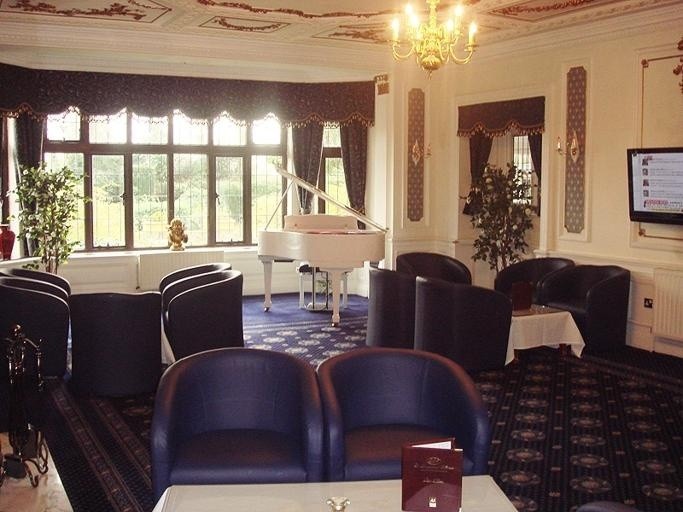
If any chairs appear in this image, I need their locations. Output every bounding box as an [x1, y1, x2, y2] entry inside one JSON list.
[[1, 261, 245, 400], [151, 346, 321, 499], [317, 347, 490, 482], [366, 250, 631, 375]]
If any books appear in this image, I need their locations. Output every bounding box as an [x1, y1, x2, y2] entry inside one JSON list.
[[400, 438, 463, 511]]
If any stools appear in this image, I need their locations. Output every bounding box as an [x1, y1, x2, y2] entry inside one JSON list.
[[297, 261, 349, 309]]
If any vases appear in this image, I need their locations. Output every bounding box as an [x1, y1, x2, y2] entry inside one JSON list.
[[0, 223, 17, 260]]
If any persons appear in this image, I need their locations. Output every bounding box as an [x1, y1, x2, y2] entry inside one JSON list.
[[167, 218, 188, 247]]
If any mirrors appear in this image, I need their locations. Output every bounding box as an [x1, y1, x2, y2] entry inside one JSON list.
[[475, 132, 539, 212]]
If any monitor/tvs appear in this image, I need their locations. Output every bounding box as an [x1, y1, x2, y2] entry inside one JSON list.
[[626, 147, 683, 224]]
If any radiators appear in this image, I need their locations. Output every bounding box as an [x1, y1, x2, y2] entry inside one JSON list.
[[653, 263, 683, 346]]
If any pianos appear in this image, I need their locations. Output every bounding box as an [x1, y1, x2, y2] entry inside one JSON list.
[[257, 163, 387, 327]]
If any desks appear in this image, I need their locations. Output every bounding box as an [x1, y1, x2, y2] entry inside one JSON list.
[[150, 474, 518, 512]]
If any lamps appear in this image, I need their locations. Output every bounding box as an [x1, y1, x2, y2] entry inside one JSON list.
[[385, 0, 479, 80], [407, 142, 432, 163], [551, 135, 578, 156]]
